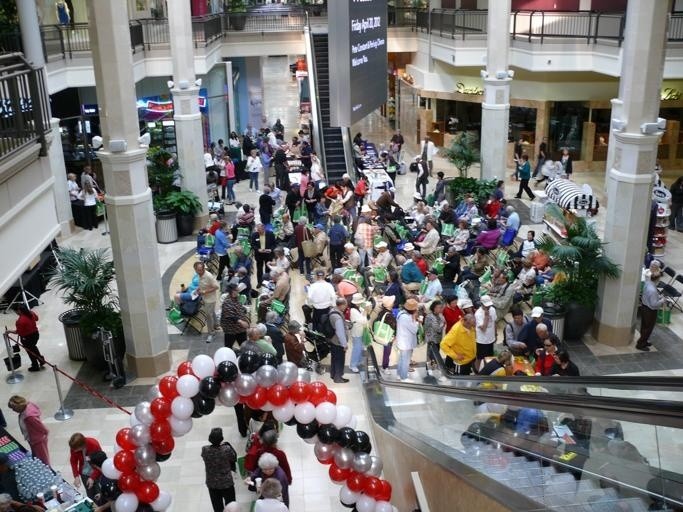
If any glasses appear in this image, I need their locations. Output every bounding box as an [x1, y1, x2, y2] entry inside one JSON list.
[[543, 344, 552, 347]]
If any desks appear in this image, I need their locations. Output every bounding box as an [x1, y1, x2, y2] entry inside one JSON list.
[[0, 431, 95, 511]]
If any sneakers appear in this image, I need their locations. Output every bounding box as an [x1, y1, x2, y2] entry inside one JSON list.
[[635, 341, 652, 351], [349, 365, 359, 372], [206, 335, 216, 342]]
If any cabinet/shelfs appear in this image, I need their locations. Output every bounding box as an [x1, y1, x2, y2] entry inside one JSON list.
[[362, 140, 393, 213]]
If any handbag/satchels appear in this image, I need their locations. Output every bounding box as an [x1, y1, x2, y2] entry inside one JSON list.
[[420, 283, 428, 295], [388, 225, 408, 244], [374, 320, 394, 347], [248, 475, 263, 493], [271, 299, 285, 315], [206, 226, 251, 263], [417, 326, 425, 346], [363, 326, 371, 346], [657, 306, 671, 325], [236, 456, 251, 477], [441, 223, 456, 237], [497, 343, 509, 357], [169, 305, 184, 325], [320, 311, 336, 339], [479, 250, 510, 296], [292, 207, 309, 223], [432, 259, 444, 274], [302, 239, 316, 257], [238, 294, 248, 304]]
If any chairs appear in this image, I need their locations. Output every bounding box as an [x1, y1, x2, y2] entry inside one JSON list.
[[652, 259, 683, 313]]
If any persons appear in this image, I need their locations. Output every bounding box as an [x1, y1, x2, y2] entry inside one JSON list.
[[65, 0, 74, 33], [55, 0, 72, 38], [0, 115, 683, 512]]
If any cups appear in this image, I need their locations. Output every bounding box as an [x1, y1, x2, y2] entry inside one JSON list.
[[419, 316, 424, 320], [254, 477, 262, 487], [36, 492, 47, 505]]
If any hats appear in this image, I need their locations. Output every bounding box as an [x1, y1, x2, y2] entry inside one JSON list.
[[531, 306, 544, 318], [351, 293, 364, 305], [344, 266, 387, 285], [480, 295, 493, 307], [288, 320, 301, 332], [413, 192, 423, 200], [314, 224, 325, 230], [375, 241, 388, 248], [382, 295, 396, 309], [403, 243, 414, 251], [403, 299, 418, 311], [361, 204, 372, 212], [461, 299, 474, 310]]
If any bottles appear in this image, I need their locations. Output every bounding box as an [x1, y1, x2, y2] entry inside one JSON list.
[[13, 456, 63, 503]]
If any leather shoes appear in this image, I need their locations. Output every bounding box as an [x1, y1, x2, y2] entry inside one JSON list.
[[335, 377, 349, 383]]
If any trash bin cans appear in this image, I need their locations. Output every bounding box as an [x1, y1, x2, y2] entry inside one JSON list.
[[58, 309, 87, 363], [154, 208, 178, 244]]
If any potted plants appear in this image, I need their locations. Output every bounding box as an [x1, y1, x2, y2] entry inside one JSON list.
[[538, 200, 620, 341], [443, 127, 497, 209], [145, 146, 203, 243], [228, 7, 247, 30], [41, 246, 116, 361]]
